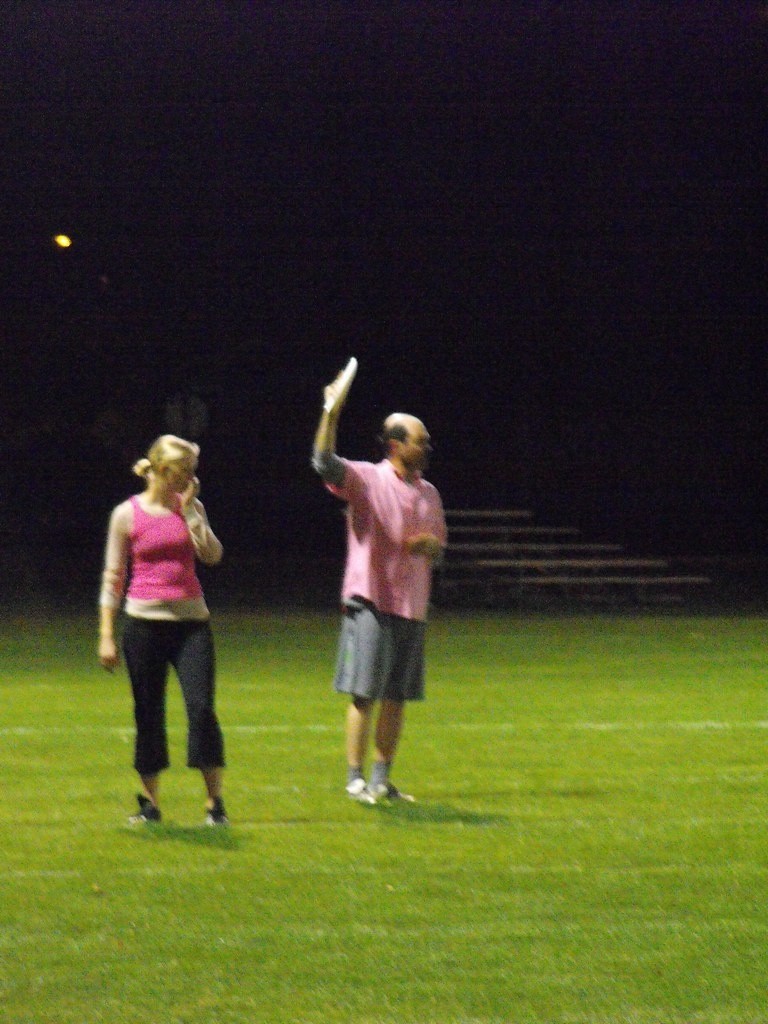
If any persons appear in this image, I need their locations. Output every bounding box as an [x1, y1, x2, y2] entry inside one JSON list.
[[97, 435, 230, 825], [313, 371, 446, 809]]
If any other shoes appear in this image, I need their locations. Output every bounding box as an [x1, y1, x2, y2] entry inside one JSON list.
[[346, 778, 377, 805], [206, 808, 228, 827], [367, 779, 414, 803], [127, 794, 160, 830]]
